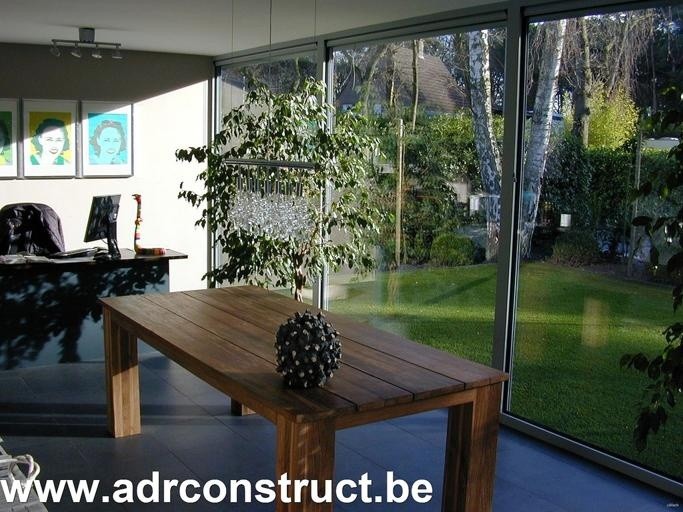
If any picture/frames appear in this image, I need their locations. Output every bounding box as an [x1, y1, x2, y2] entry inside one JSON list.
[[0, 95, 134, 180]]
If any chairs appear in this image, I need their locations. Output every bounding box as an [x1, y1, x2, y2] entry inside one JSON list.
[[0, 203, 62, 255]]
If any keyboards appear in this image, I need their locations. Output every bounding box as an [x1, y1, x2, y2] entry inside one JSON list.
[[52, 248, 95, 258]]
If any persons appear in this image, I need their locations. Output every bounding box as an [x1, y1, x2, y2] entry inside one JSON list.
[[89, 119, 128, 164], [30, 118, 70, 165]]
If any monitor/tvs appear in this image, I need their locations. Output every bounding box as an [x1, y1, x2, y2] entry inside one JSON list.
[[85, 194, 121, 259]]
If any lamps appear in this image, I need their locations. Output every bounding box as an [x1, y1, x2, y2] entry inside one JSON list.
[[49, 27, 123, 59], [225, 0, 320, 241]]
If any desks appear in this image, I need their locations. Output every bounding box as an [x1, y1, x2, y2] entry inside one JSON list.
[[99, 284, 510, 512], [0, 248, 188, 371]]
